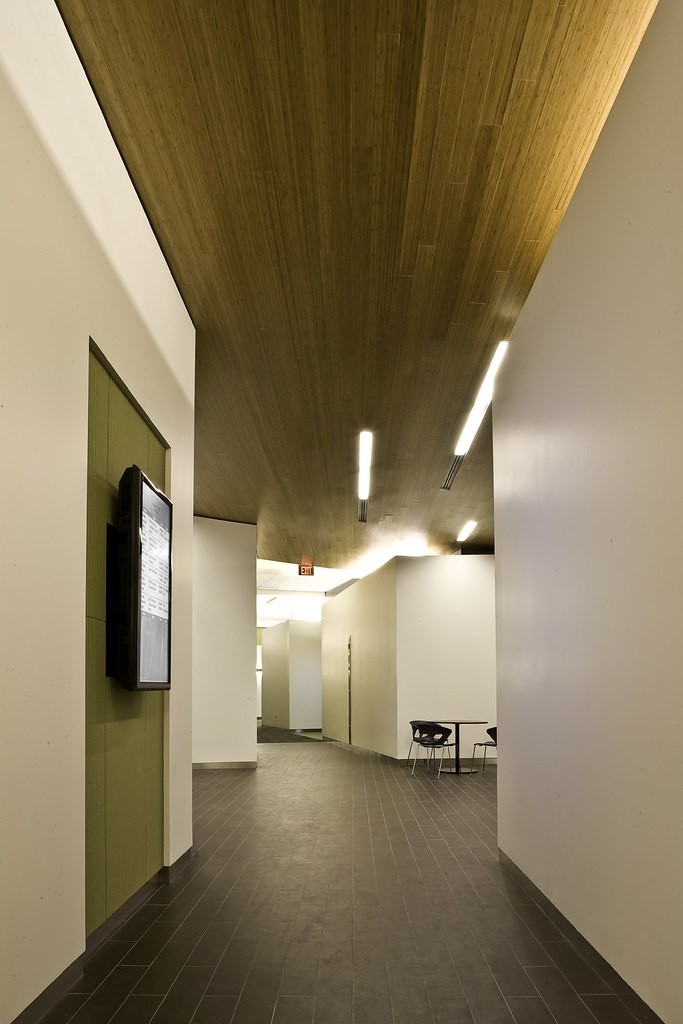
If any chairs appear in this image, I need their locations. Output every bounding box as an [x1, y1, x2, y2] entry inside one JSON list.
[[470, 727, 497, 776], [406, 720, 463, 779]]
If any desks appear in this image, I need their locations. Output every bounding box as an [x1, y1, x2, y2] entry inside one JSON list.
[[428, 721, 488, 774]]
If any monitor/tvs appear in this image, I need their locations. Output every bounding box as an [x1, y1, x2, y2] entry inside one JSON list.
[[113, 464, 173, 692]]
